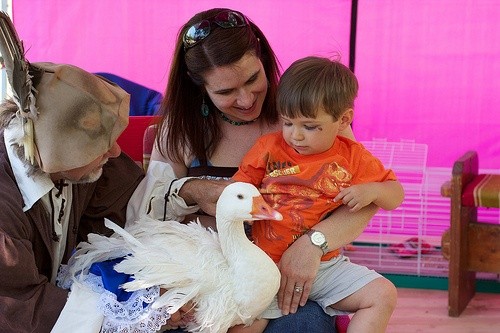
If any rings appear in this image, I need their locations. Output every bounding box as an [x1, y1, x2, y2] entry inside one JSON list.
[[294, 286, 303, 293]]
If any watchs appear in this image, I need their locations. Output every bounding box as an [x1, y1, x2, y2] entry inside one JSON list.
[[303, 229, 329, 256]]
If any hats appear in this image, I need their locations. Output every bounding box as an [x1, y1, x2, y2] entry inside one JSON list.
[[0, 12, 130, 172]]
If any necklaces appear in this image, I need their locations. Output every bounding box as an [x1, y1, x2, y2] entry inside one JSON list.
[[219, 112, 260, 126]]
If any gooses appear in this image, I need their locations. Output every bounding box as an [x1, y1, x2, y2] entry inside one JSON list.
[[58, 181, 284, 333]]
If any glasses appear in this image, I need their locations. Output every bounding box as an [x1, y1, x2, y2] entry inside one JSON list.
[[181, 10, 250, 51]]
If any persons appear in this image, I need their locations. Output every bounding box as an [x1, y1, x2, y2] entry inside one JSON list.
[[0, 12, 235, 333], [230, 56, 404, 333], [148, 7, 379, 333]]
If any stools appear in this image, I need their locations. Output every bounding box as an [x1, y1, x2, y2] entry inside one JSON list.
[[442, 150, 500, 317]]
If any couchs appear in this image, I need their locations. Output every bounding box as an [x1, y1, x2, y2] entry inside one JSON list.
[[116, 115, 351, 333]]
